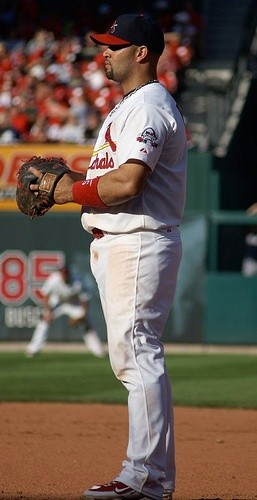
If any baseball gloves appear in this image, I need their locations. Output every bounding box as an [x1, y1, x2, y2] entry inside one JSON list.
[[15, 155, 69, 219]]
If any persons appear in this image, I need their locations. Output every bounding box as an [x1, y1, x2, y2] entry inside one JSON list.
[[30, 14, 188, 500], [26, 264, 105, 360], [0, 0, 201, 145]]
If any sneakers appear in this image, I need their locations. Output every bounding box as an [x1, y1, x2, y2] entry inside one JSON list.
[[83, 480, 172, 500]]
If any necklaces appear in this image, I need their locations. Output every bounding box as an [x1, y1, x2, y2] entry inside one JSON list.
[[109, 79, 159, 118]]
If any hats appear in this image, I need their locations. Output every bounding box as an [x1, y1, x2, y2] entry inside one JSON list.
[[90, 14, 165, 55]]
